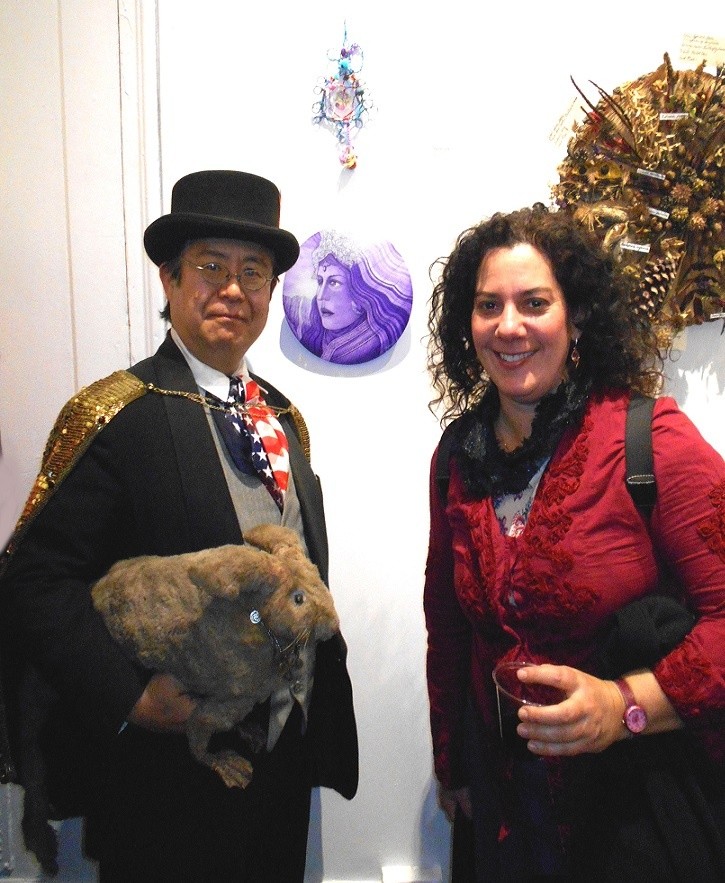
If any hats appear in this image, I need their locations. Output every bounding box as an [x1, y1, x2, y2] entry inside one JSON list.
[[143, 169, 301, 277]]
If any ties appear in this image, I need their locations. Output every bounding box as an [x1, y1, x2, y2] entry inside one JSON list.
[[227, 376, 286, 509]]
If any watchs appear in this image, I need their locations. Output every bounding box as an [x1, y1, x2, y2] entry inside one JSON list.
[[613, 679, 648, 737]]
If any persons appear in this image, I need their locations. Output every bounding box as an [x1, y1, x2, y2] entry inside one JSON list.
[[422, 203, 725, 882], [0, 169, 361, 883]]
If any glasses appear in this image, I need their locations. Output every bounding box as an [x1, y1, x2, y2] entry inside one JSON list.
[[178, 251, 277, 292]]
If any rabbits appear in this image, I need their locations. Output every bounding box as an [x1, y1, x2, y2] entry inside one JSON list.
[[16, 523, 340, 880]]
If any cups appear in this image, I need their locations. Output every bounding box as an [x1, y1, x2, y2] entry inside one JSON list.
[[491, 661, 567, 753]]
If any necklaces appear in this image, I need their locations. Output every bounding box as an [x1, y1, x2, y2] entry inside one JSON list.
[[494, 423, 511, 453]]
